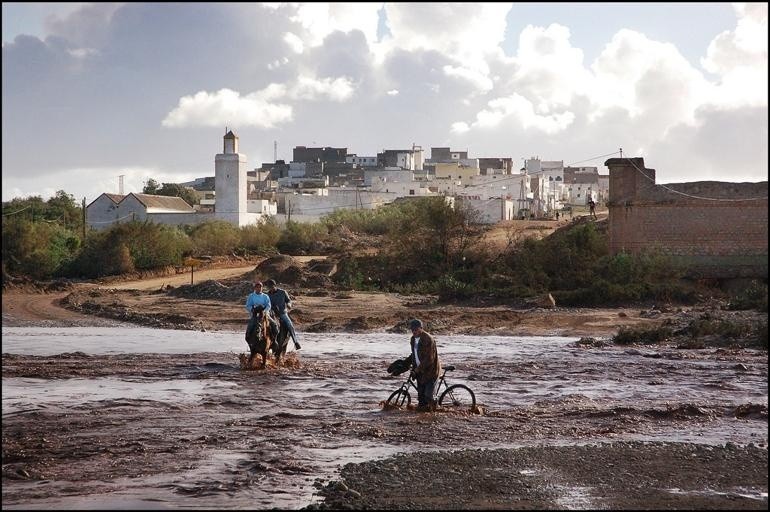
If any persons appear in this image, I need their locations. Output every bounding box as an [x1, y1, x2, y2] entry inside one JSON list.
[[246, 281, 279, 346], [587, 197, 595, 216], [556, 211, 559, 219], [266, 278, 301, 350], [404, 318, 441, 407]]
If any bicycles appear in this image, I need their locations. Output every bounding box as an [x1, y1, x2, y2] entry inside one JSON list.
[[383, 364, 477, 414]]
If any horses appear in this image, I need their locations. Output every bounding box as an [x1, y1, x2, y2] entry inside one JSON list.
[[245, 304, 280, 368], [271, 304, 291, 366]]
